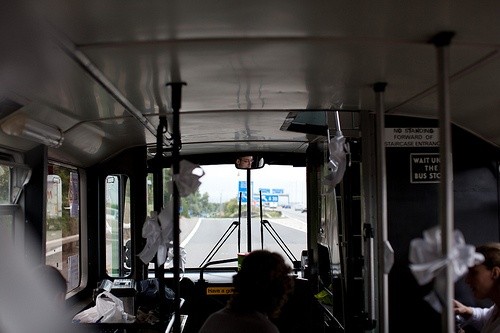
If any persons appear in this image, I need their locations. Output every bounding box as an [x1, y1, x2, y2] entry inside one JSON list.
[[198, 251, 294, 333], [0, 263, 103, 333], [454, 242, 500, 333], [236, 156, 253, 168]]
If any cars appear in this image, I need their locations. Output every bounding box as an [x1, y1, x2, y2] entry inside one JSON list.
[[264, 201, 307, 213]]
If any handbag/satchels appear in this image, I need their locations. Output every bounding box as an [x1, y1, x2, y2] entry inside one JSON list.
[[73, 291, 137, 323]]
